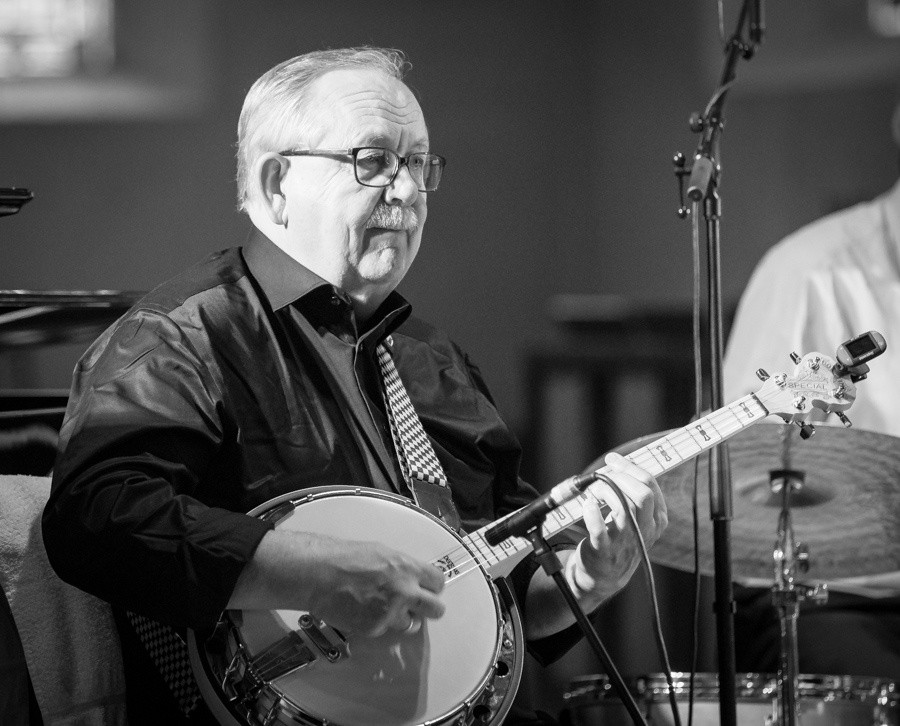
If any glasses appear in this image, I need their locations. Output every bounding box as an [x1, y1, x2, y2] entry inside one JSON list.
[[278, 146, 447, 192]]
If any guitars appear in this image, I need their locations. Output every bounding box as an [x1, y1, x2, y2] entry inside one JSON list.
[[177, 325, 893, 725]]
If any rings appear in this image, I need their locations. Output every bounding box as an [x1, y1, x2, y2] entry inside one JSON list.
[[401, 616, 414, 634]]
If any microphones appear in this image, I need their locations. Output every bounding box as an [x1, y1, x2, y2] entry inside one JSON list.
[[485, 473, 597, 546]]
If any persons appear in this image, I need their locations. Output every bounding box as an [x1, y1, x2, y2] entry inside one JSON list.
[[715, 178, 900, 680], [41, 46, 668, 726]]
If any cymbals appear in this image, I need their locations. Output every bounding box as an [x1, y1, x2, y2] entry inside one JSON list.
[[580, 416, 898, 581]]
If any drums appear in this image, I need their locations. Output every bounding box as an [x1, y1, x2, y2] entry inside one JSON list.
[[567, 666, 894, 726]]
